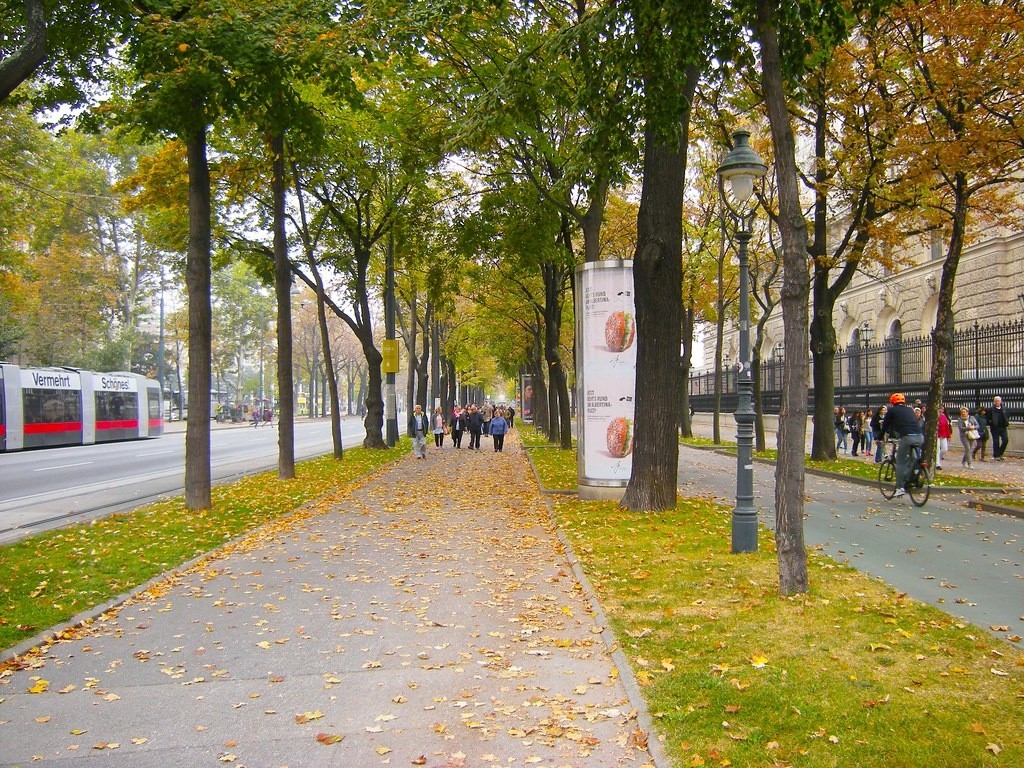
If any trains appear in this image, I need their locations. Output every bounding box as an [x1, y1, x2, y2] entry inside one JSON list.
[[0, 360, 168, 453]]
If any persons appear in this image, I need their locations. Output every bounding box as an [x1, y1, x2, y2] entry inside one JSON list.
[[431, 407, 445, 450], [688, 403, 694, 423], [215, 402, 274, 429], [525, 383, 534, 410], [450, 402, 514, 453], [360, 405, 366, 420], [832, 393, 1010, 496], [408, 405, 428, 459]]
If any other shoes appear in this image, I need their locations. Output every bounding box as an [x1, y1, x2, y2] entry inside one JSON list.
[[837, 452, 840, 454], [844, 452, 847, 454], [959, 462, 964, 468], [417, 457, 421, 460], [936, 466, 942, 470], [422, 454, 426, 459], [457, 446, 461, 449], [852, 451, 858, 456], [441, 445, 444, 449], [874, 461, 882, 465], [468, 445, 474, 450], [495, 448, 502, 452], [436, 447, 439, 450], [454, 444, 456, 447], [979, 456, 988, 462], [966, 465, 975, 470], [992, 456, 1004, 462], [484, 435, 488, 437], [865, 451, 872, 456]]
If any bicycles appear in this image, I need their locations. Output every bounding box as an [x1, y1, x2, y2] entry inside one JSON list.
[[876, 438, 930, 508]]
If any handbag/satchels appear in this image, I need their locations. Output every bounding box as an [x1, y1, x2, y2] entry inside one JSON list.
[[967, 429, 980, 440], [443, 426, 449, 434], [844, 425, 850, 433]]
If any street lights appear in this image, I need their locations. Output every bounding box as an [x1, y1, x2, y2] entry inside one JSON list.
[[861, 322, 873, 412], [723, 354, 732, 412], [715, 127, 768, 553], [689, 364, 694, 415], [776, 343, 785, 397]]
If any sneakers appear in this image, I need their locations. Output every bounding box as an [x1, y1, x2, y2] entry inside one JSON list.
[[892, 487, 906, 497]]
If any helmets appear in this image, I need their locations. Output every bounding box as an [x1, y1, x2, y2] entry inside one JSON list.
[[889, 393, 905, 405]]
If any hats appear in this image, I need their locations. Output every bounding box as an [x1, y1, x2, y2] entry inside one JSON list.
[[913, 406, 921, 412], [470, 406, 476, 410]]
